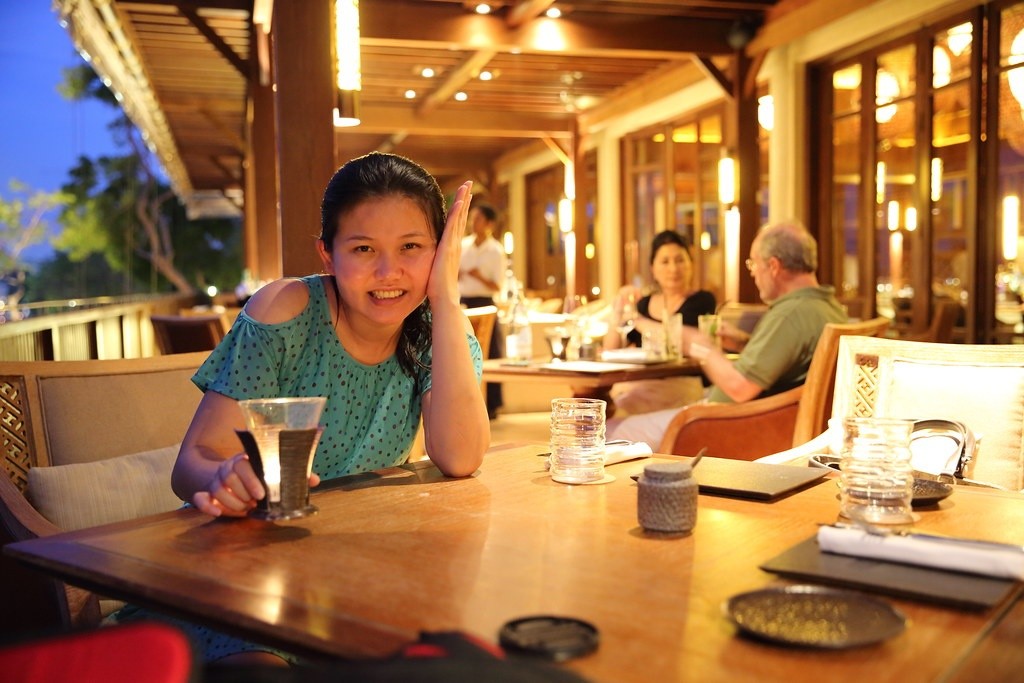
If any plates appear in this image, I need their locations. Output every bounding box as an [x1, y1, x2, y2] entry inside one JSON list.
[[728, 585, 907, 646]]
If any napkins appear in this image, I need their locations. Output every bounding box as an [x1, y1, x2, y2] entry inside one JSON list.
[[544, 441, 653, 470], [818, 524, 1024, 582]]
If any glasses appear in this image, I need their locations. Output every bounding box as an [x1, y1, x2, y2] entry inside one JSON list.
[[744, 254, 783, 271]]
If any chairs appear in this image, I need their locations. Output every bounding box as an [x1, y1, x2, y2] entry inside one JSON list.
[[462, 305, 497, 404], [0, 348, 255, 641], [715, 300, 772, 354], [660, 316, 891, 461], [147, 315, 224, 358], [752, 334, 1024, 493]]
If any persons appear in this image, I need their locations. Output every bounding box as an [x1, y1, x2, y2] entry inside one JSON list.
[[608, 215, 848, 455], [595, 230, 717, 438], [455, 202, 505, 422], [116, 153, 491, 683]]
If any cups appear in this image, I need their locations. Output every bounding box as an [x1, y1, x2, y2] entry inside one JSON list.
[[550, 396, 608, 483], [541, 310, 723, 364], [238, 396, 327, 516], [839, 418, 920, 532]]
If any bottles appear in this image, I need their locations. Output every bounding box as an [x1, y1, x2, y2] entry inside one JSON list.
[[504, 286, 533, 363], [636, 461, 699, 531]]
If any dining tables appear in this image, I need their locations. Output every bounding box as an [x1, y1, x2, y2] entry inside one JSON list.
[[0, 350, 1024, 683]]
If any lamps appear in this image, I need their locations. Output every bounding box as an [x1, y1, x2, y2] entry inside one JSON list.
[[332, 0, 362, 129], [557, 194, 572, 233], [504, 229, 514, 254]]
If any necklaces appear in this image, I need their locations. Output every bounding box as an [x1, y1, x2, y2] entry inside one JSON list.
[[663, 289, 687, 319]]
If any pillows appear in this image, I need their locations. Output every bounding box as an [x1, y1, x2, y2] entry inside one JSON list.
[[28, 442, 187, 618]]
